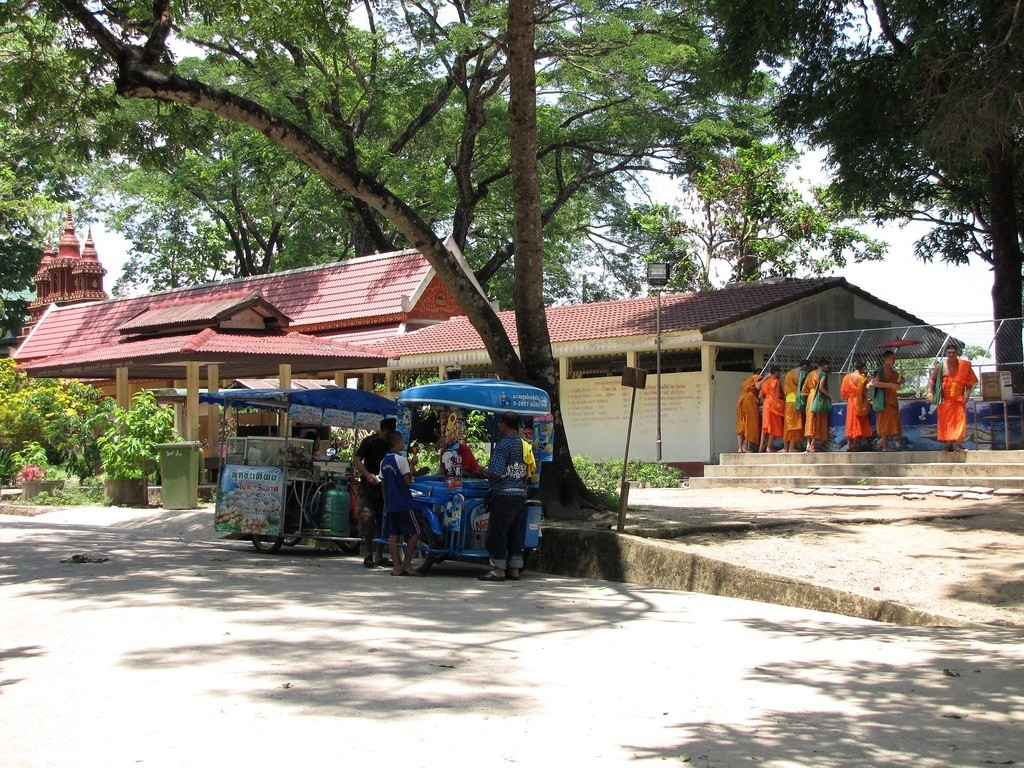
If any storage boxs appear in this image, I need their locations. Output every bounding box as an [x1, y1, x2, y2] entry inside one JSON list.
[[980, 370, 1014, 401]]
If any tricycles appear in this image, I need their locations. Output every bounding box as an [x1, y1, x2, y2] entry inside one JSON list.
[[196, 386, 395, 556], [367, 377, 554, 576]]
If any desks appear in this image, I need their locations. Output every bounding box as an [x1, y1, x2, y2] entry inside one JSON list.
[[973, 395, 1024, 450]]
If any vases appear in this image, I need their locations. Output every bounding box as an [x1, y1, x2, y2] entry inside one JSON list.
[[20, 479, 65, 502]]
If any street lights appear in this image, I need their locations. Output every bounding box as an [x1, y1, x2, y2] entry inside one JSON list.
[[646, 261, 672, 465]]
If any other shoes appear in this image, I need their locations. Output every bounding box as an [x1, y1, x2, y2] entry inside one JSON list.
[[364, 557, 375, 566], [375, 557, 394, 568], [737, 443, 969, 453]]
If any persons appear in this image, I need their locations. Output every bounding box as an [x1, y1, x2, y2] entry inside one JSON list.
[[378, 429, 425, 578], [866, 351, 906, 452], [478, 410, 530, 582], [926, 345, 979, 452], [735, 359, 838, 453], [354, 417, 397, 568], [440, 443, 488, 477], [840, 359, 872, 451], [304, 432, 328, 461]]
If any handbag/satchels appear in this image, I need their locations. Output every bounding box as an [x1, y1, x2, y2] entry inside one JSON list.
[[854, 374, 871, 416], [868, 367, 885, 411], [484, 485, 528, 514], [931, 360, 943, 405], [766, 379, 785, 417], [795, 370, 807, 410], [809, 372, 832, 414]]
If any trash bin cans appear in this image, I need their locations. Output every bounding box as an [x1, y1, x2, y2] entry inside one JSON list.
[[154, 441, 204, 509]]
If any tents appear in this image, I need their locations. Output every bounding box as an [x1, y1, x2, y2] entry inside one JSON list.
[[200, 387, 398, 470]]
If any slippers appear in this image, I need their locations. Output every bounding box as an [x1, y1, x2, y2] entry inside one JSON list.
[[403, 569, 424, 577], [390, 572, 405, 576], [478, 572, 507, 582], [505, 570, 518, 580]]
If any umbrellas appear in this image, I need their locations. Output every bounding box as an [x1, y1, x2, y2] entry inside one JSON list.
[[875, 336, 924, 379]]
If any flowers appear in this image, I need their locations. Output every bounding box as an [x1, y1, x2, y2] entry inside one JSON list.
[[16, 463, 48, 482]]
